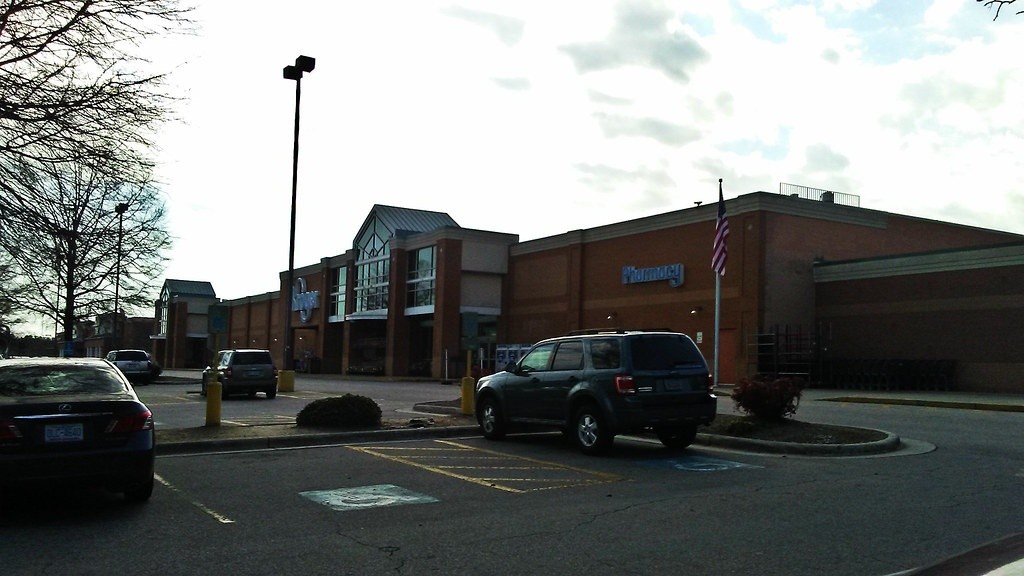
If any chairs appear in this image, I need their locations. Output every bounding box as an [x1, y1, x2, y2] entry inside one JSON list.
[[0, 379, 25, 395], [83, 376, 108, 391]]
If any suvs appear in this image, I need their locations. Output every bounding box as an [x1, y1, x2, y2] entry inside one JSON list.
[[201, 349, 278, 399], [474, 327, 717, 459], [104, 349, 154, 386]]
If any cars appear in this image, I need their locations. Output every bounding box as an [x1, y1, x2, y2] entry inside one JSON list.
[[0, 352, 156, 502]]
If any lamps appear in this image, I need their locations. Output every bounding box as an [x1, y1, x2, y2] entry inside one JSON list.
[[607, 313, 617, 320], [691, 306, 702, 314]]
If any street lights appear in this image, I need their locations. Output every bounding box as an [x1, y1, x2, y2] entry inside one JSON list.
[[282, 54, 316, 371], [114, 202, 130, 350]]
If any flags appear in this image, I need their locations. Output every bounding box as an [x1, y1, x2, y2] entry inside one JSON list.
[[709, 188, 731, 277]]
